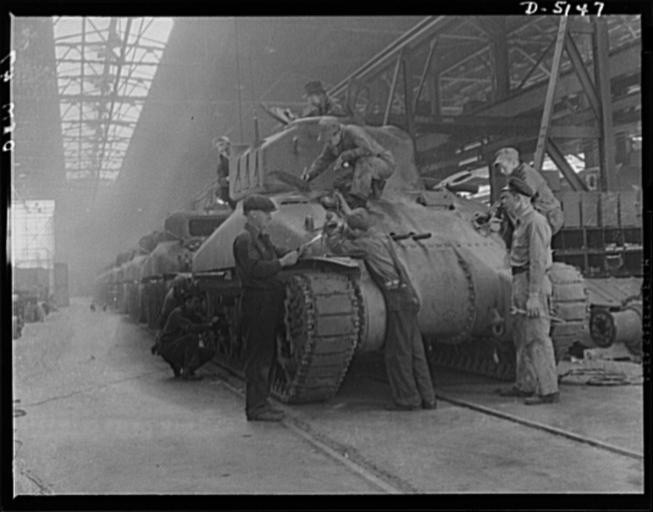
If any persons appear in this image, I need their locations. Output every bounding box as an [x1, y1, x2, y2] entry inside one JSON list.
[[233, 194, 313, 423], [326, 190, 437, 409], [485, 148, 563, 241], [298, 117, 394, 210], [160, 287, 221, 383], [156, 273, 194, 331], [213, 134, 237, 210], [300, 78, 351, 119], [501, 177, 560, 404]]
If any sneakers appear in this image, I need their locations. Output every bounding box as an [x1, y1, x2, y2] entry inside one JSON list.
[[500, 386, 532, 397], [248, 413, 283, 421], [372, 179, 385, 200], [165, 359, 180, 377], [264, 404, 284, 413], [347, 195, 367, 210], [526, 392, 559, 405], [183, 371, 201, 380], [421, 399, 437, 410], [383, 401, 421, 411]]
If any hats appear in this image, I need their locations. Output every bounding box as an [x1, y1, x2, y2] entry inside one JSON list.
[[302, 80, 324, 97], [316, 116, 339, 143], [243, 194, 278, 211], [500, 178, 533, 196], [494, 147, 519, 168]]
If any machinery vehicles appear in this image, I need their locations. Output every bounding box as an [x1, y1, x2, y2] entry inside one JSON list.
[[91, 104, 594, 404]]
